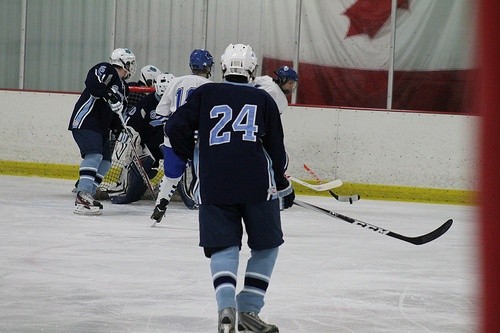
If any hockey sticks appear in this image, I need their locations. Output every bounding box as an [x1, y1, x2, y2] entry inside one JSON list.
[[283, 173, 343, 192], [286, 147, 361, 202], [118, 112, 159, 200], [293, 198, 454, 246]]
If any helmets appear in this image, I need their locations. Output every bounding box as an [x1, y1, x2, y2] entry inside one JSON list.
[[110, 48, 137, 80], [273, 66, 299, 85], [140, 65, 161, 87], [220, 44, 258, 81], [189, 48, 214, 74], [154, 72, 176, 98]]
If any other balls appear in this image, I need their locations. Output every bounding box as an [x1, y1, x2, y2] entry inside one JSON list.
[[348, 198, 353, 204]]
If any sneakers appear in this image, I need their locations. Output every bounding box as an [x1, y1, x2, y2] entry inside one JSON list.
[[73, 193, 103, 216], [150, 205, 168, 225], [237, 311, 280, 333], [217, 306, 237, 333]]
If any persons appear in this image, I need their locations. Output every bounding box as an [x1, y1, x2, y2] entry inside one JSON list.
[[67, 48, 299, 223], [165, 44, 295, 333]]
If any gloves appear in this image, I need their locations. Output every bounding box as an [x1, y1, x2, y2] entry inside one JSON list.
[[276, 177, 296, 210], [103, 88, 123, 113]]
